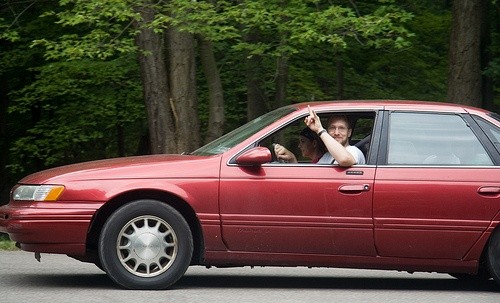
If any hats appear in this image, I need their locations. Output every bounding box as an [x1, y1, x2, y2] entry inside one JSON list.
[[300, 127, 315, 140]]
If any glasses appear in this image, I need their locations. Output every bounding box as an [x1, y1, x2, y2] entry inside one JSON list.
[[328, 128, 349, 132]]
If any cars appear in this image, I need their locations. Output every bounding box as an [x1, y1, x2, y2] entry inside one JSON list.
[[0, 99, 500, 290]]
